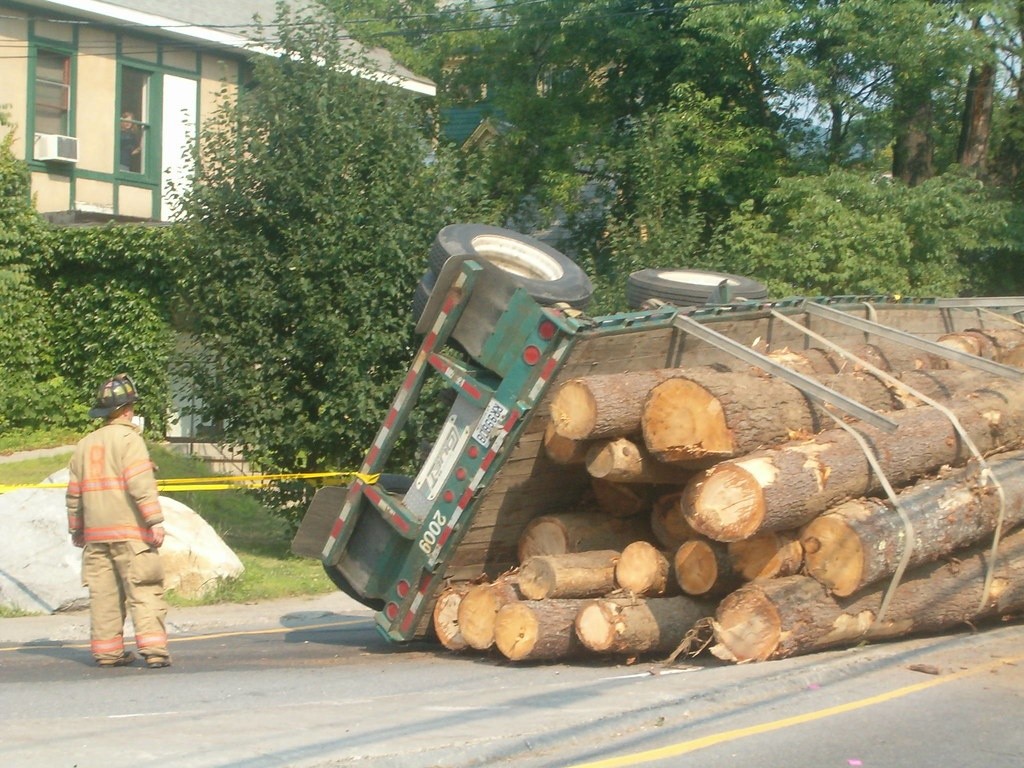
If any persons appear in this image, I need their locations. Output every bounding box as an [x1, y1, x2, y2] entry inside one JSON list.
[[66, 373, 172, 669]]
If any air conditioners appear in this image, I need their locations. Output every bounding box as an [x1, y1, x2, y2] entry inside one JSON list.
[[38, 134, 80, 164]]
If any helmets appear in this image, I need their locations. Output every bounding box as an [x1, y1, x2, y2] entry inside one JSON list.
[[88, 372, 138, 418]]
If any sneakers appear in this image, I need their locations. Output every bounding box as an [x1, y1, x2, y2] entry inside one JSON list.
[[98, 651, 135, 666], [145, 655, 173, 667]]
